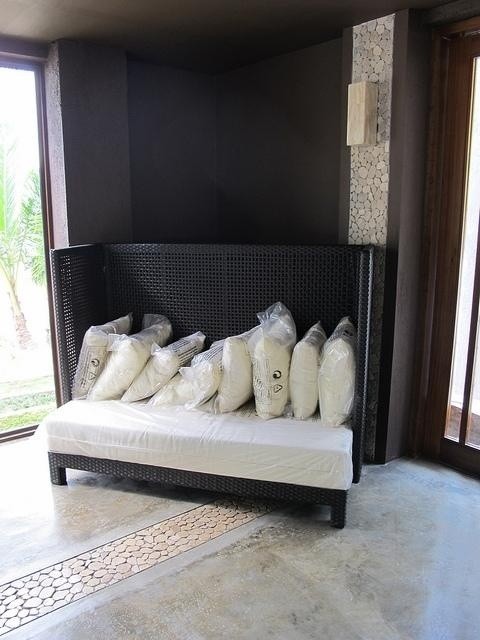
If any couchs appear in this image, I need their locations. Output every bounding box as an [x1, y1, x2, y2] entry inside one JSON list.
[[30, 241, 375, 531]]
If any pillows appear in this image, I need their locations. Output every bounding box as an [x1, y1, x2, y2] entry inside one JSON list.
[[71, 314, 358, 426]]
